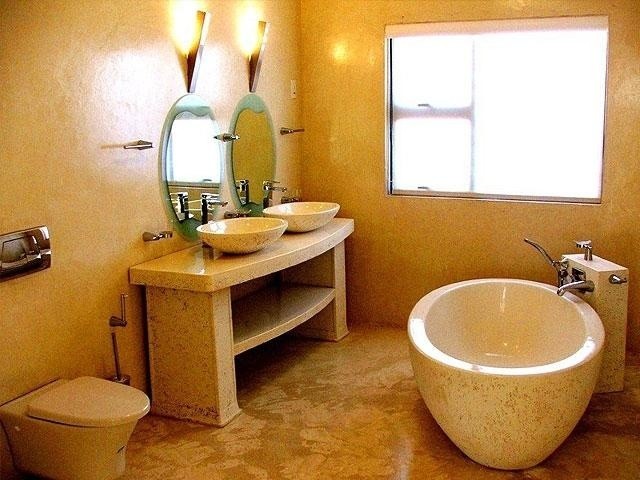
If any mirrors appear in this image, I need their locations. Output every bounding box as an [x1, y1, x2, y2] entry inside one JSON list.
[[227, 94, 277, 216], [161, 94, 224, 242]]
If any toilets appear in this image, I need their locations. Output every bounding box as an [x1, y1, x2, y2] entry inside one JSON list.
[[0, 376, 151, 479]]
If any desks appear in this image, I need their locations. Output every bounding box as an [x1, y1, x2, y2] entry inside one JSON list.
[[129, 218, 354, 428]]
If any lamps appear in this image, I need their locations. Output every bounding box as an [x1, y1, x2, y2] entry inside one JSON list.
[[231, 0, 271, 92], [163, 0, 211, 93]]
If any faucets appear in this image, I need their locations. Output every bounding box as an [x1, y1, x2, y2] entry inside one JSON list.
[[235, 179, 249, 204], [169, 192, 189, 220], [261, 181, 287, 209], [557, 280, 594, 296], [200, 193, 228, 224]]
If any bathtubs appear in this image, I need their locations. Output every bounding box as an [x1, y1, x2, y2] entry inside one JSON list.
[[407, 277, 607, 471]]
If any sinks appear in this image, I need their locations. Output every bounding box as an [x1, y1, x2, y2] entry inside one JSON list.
[[262, 202, 340, 233], [196, 217, 288, 255], [189, 200, 201, 220]]
